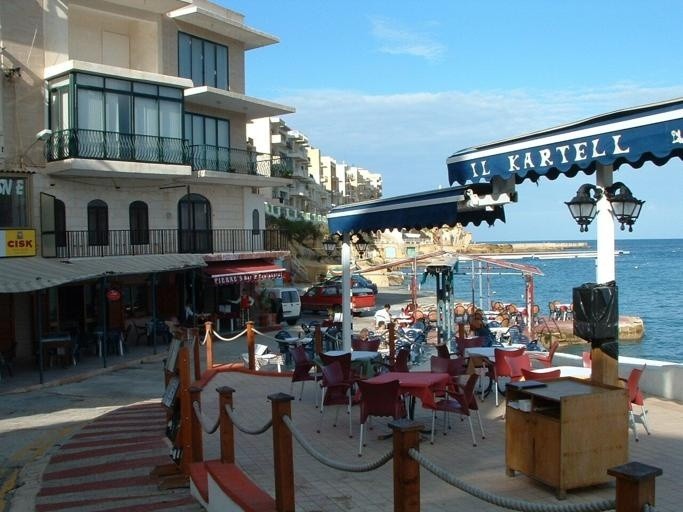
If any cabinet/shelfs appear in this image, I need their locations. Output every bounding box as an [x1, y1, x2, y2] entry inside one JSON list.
[[502, 373, 628, 501]]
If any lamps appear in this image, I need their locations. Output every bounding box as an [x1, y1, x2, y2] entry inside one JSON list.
[[350, 231, 368, 258], [322, 232, 342, 256], [604, 180, 646, 232], [562, 182, 602, 233], [17, 127, 54, 167]]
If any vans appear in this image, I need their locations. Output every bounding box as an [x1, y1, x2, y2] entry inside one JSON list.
[[255, 287, 301, 325]]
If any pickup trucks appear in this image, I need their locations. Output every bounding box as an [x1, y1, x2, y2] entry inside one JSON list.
[[299, 286, 375, 316]]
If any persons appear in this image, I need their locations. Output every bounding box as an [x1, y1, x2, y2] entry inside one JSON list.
[[170, 288, 495, 362]]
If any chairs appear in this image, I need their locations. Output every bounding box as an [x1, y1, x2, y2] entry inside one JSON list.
[[29, 297, 241, 373]]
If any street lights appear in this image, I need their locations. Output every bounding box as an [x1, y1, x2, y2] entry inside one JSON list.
[[322, 233, 368, 352], [564, 180, 646, 387]]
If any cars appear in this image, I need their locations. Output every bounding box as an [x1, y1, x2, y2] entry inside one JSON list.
[[316, 274, 377, 294]]
